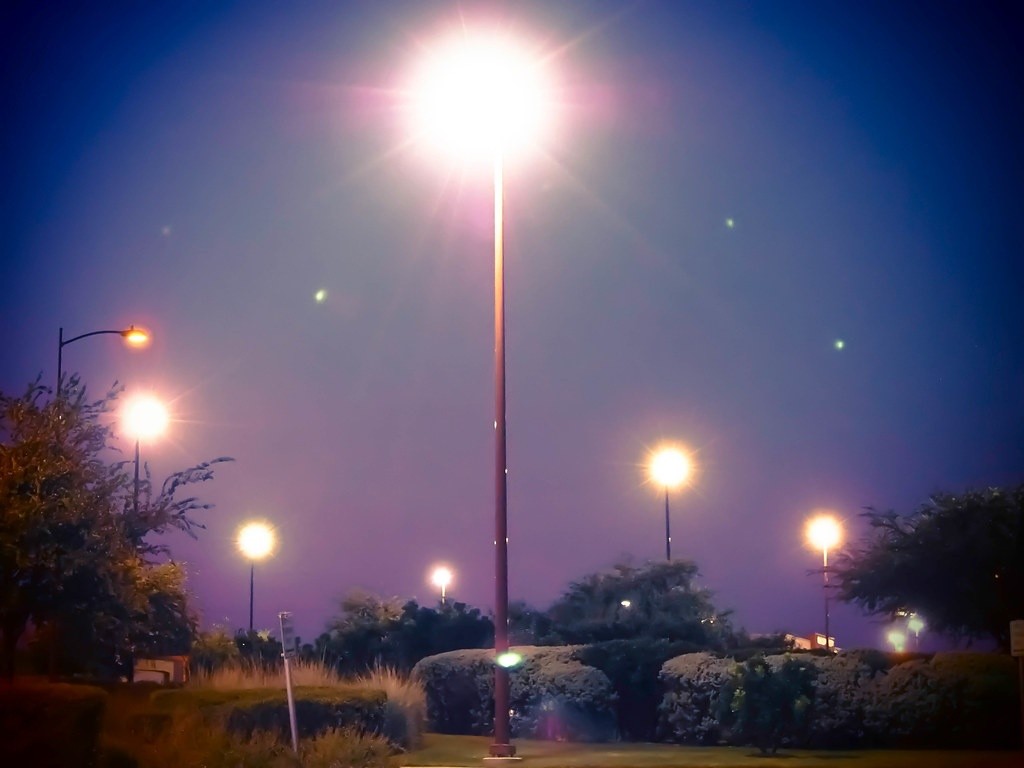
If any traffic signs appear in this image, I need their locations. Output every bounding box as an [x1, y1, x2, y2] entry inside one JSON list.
[[1010, 621, 1024, 656]]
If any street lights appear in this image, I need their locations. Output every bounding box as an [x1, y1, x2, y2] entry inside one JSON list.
[[110, 392, 169, 553], [234, 520, 278, 636], [400, 21, 567, 758], [53, 324, 148, 408], [645, 442, 692, 563], [429, 566, 453, 608], [806, 512, 843, 651]]
